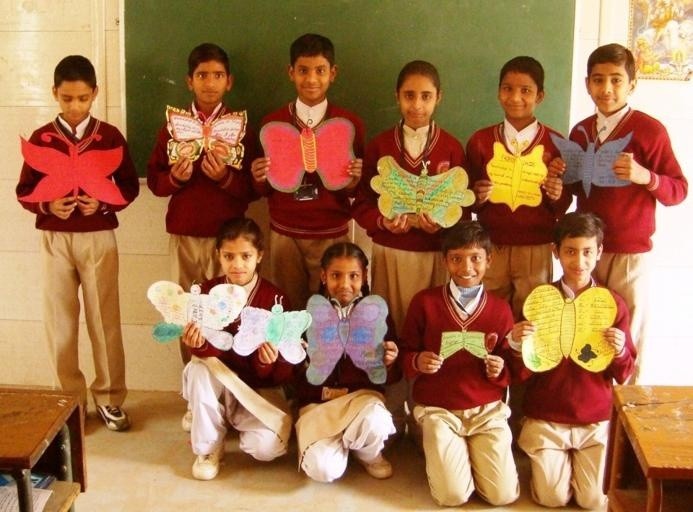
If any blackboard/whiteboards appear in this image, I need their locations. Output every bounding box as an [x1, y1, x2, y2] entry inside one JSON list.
[[120, 0, 582, 184]]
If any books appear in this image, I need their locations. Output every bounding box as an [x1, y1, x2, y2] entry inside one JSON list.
[[0, 471, 81, 510]]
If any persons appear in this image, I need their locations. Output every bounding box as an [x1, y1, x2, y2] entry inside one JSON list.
[[288, 242, 399, 487], [250, 32, 367, 437], [178, 215, 289, 485], [13, 57, 141, 431], [146, 42, 256, 429], [547, 42, 687, 386], [466, 55, 576, 322], [351, 60, 469, 445], [399, 220, 521, 504], [501, 211, 638, 511]]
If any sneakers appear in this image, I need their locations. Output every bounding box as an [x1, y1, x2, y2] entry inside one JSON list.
[[192, 444, 224, 483], [362, 454, 398, 480], [92, 400, 131, 433]]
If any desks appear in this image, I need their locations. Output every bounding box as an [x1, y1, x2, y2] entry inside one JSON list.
[[600, 385, 692, 511], [0, 383, 88, 512]]
